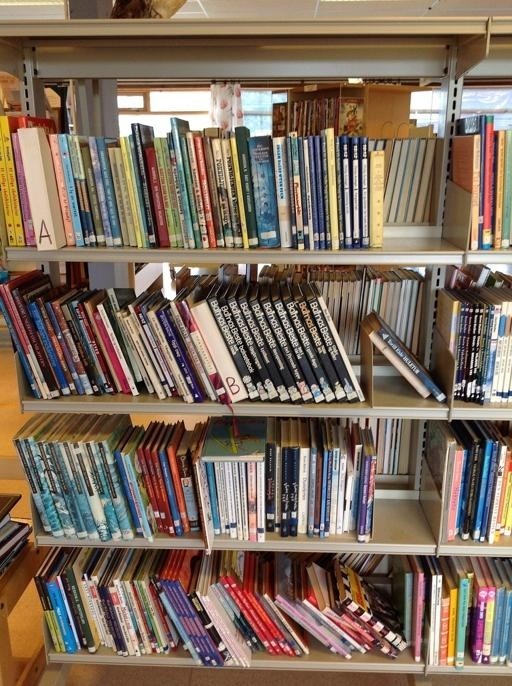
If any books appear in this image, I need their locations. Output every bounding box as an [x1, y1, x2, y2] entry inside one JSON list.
[[419, 422, 512, 545], [0, 266, 366, 403], [13, 414, 418, 545], [0, 115, 384, 251], [365, 263, 445, 405], [366, 137, 436, 226], [432, 265, 511, 406], [450, 114, 511, 250], [273, 97, 363, 139], [0, 493, 32, 579], [33, 545, 512, 670]]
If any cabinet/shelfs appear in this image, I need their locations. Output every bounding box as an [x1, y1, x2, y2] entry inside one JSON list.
[[0, 14, 512, 686]]
